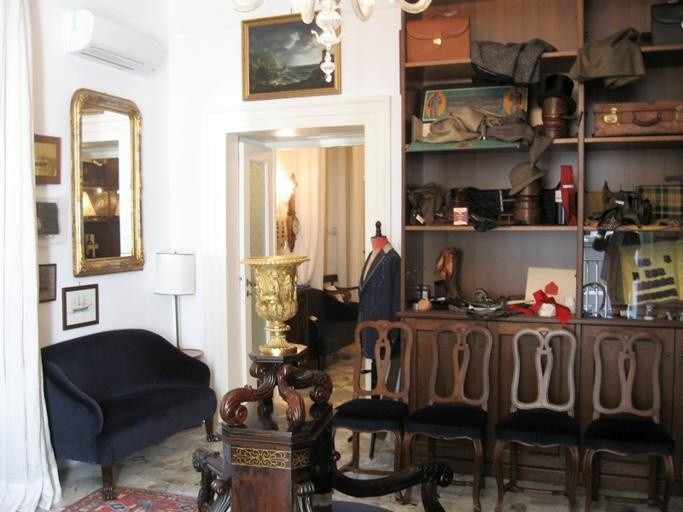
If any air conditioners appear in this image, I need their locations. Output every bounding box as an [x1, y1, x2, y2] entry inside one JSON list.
[[70, 8, 157, 72]]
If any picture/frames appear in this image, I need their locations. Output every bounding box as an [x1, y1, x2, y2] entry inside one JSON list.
[[62, 283, 99, 330], [240, 6, 342, 102], [34, 134, 62, 185], [422, 85, 530, 122], [39, 264, 57, 302]]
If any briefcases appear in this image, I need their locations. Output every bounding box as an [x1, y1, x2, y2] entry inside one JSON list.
[[590, 99, 682, 138]]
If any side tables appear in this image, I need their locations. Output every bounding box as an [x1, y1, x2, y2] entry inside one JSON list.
[[180, 348, 203, 358]]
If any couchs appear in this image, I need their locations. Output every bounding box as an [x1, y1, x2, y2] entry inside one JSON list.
[[40, 329, 217, 501], [284, 288, 360, 371]]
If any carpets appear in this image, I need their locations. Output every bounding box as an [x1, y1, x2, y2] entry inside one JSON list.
[[60, 485, 199, 512]]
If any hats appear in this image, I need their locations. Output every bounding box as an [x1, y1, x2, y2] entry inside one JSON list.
[[537, 73, 579, 117], [418, 115, 479, 144], [504, 161, 550, 196]]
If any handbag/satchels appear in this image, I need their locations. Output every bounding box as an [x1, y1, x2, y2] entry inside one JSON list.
[[650, 2, 683, 45], [404, 13, 473, 63]]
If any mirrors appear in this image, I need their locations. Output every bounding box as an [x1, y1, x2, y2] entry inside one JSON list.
[[70, 89, 144, 277]]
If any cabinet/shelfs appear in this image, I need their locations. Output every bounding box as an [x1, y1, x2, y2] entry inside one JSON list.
[[397, 0, 683, 321], [581, 325, 683, 481], [403, 318, 576, 470]]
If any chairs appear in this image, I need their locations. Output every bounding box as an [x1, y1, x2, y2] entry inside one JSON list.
[[323, 274, 359, 305], [193, 402, 453, 512], [583, 332, 675, 512], [492, 326, 581, 512], [400, 321, 493, 512], [332, 319, 416, 502]]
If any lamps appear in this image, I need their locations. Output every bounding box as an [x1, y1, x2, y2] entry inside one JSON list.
[[224, 0, 436, 85], [153, 252, 204, 358]]
